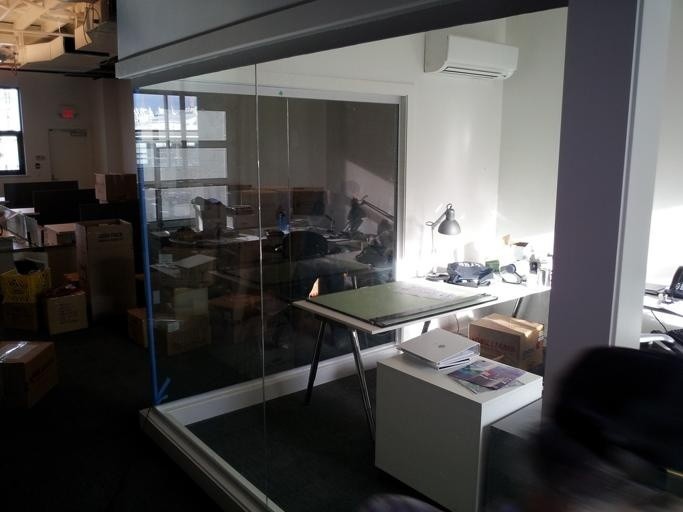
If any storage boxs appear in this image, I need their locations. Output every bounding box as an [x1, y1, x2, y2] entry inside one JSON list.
[[148, 188, 327, 356], [0, 173, 151, 410], [468, 313, 544, 370]]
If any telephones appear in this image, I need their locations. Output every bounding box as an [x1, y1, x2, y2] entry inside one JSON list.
[[669, 266, 683, 299]]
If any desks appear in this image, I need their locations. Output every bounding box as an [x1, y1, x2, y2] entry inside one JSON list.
[[294, 279, 549, 437], [209, 249, 380, 354], [375, 355, 538, 511]]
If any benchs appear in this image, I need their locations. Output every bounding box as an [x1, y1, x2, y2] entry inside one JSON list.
[[529, 346, 683, 512]]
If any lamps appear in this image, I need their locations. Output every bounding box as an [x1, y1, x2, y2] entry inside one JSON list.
[[347, 195, 396, 265], [425, 203, 461, 280]]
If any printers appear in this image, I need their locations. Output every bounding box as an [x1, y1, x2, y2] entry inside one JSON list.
[[226, 205, 258, 230]]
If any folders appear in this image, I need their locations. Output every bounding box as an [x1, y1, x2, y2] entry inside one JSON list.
[[395, 327, 482, 372]]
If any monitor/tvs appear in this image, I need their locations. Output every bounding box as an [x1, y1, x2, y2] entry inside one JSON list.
[[292, 187, 328, 219]]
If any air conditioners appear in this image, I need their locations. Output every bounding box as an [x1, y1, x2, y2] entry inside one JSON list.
[[424, 31, 519, 80]]
